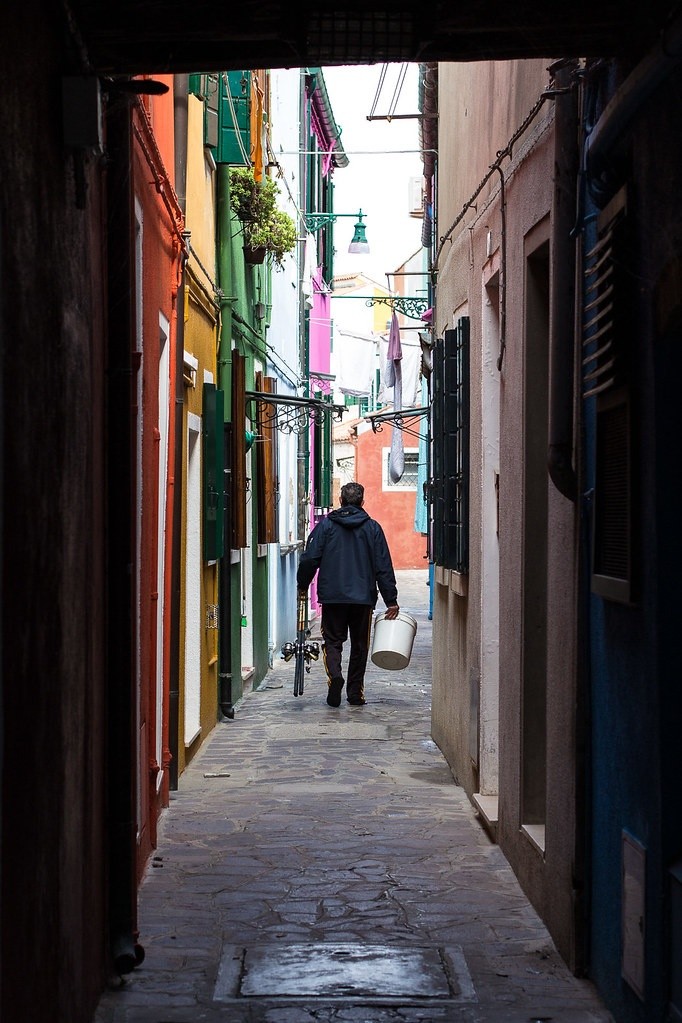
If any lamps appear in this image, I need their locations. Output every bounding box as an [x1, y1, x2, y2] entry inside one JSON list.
[[305, 208, 370, 254]]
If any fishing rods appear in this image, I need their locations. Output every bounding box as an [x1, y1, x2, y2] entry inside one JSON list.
[[281, 490, 320, 697]]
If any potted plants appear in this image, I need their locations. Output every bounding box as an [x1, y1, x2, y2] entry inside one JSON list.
[[228, 167, 301, 272]]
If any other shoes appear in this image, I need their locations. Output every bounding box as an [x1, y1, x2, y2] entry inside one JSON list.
[[327, 676, 344, 707], [348, 690, 366, 705]]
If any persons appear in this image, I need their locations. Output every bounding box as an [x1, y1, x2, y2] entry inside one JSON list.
[[297, 483, 399, 707]]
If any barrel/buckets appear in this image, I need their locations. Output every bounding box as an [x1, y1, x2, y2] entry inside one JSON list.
[[371, 611, 417, 670]]
